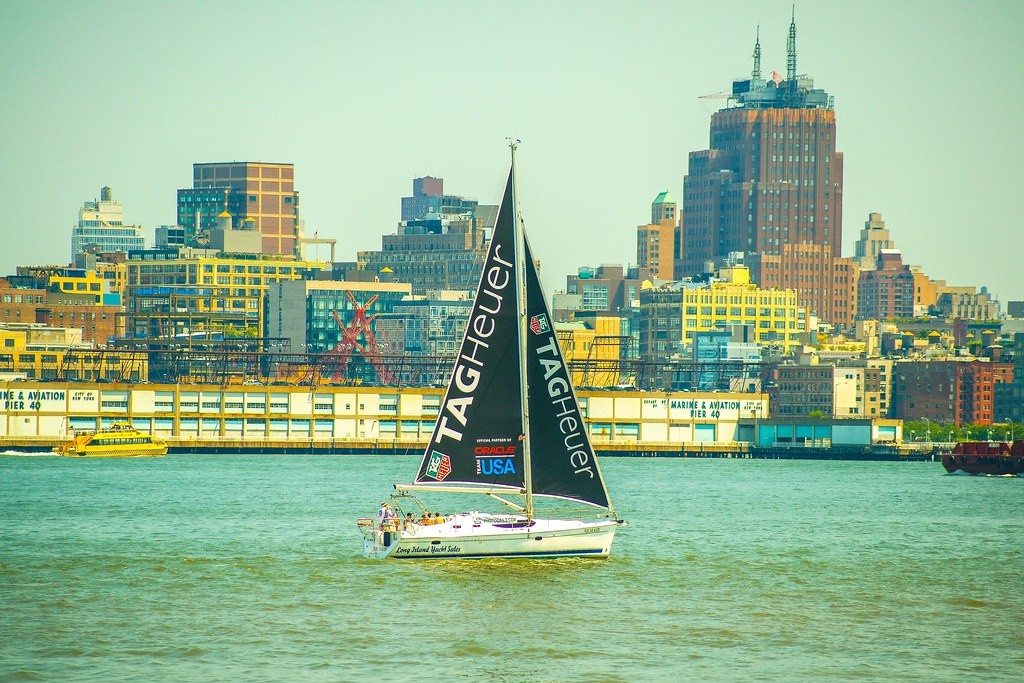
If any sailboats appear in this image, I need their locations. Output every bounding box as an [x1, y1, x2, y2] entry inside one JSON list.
[[353, 134, 626, 564]]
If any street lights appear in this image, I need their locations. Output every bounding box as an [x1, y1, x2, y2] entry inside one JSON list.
[[987, 431, 993, 441], [921, 417, 930, 443], [1005, 417, 1014, 443], [925, 431, 931, 443], [909, 430, 915, 443], [948, 431, 954, 443], [966, 430, 972, 440], [1005, 431, 1010, 441]]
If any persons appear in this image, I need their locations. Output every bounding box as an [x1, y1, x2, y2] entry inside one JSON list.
[[377, 502, 444, 531]]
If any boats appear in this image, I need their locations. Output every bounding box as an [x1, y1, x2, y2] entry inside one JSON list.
[[941, 439, 1024, 474], [51, 417, 169, 457]]
